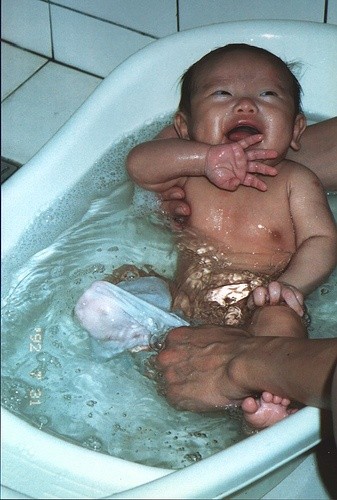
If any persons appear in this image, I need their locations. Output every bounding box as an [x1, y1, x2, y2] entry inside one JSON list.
[[126, 41, 335, 435], [147, 111, 336, 495]]
